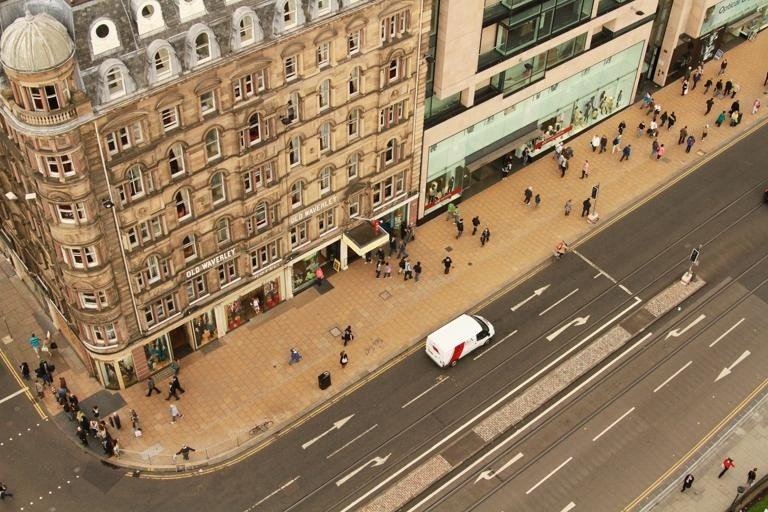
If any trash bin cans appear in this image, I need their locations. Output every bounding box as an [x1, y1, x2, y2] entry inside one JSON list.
[[318, 371, 331, 390]]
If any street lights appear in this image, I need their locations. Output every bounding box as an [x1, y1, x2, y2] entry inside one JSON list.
[[0, 311, 13, 338]]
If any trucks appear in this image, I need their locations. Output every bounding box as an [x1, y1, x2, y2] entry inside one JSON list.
[[425, 312, 494, 368]]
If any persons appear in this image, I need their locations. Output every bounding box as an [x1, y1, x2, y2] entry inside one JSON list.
[[480, 228, 490, 246], [582, 198, 591, 217], [682, 58, 742, 139], [17, 331, 196, 464], [565, 199, 572, 216], [250, 267, 359, 369], [472, 216, 480, 235], [554, 240, 567, 259], [522, 147, 529, 166], [581, 159, 589, 179], [591, 134, 607, 154], [552, 142, 573, 177], [374, 220, 421, 282], [745, 466, 757, 487], [681, 472, 695, 493], [428, 184, 436, 203], [448, 176, 454, 193], [446, 203, 464, 239], [613, 92, 695, 162], [502, 155, 512, 177], [752, 98, 760, 114], [572, 91, 622, 125], [442, 256, 452, 274], [523, 186, 541, 207], [716, 457, 735, 481]]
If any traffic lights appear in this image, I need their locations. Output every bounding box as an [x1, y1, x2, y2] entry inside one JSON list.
[[591, 186, 597, 200], [690, 248, 699, 264]]
